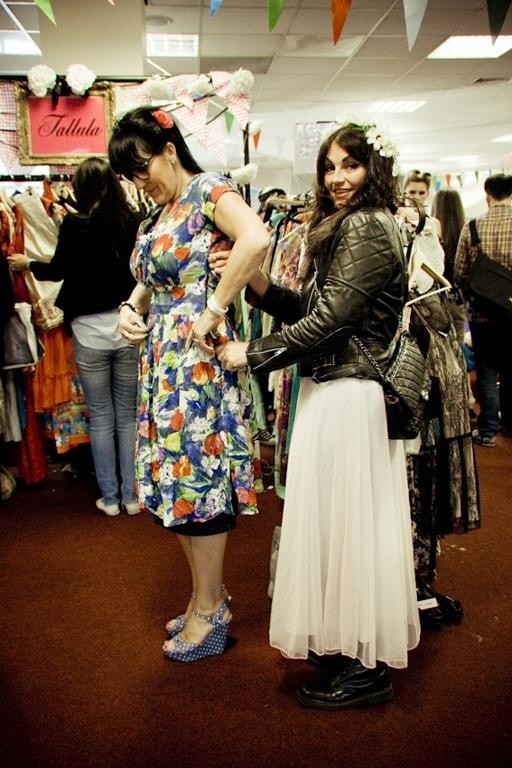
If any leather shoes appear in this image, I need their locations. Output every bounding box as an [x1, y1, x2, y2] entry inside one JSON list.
[[417, 592, 444, 627], [294, 659, 394, 711], [423, 587, 463, 621]]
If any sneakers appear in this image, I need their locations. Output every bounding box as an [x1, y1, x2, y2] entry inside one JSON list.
[[95, 497, 121, 516], [256, 429, 277, 446], [124, 501, 141, 516], [471, 429, 496, 447]]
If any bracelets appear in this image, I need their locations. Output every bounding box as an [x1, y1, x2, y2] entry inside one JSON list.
[[206, 295, 230, 317], [26, 258, 38, 272], [117, 301, 138, 315]]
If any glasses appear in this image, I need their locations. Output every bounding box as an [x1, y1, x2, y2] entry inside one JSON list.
[[119, 153, 155, 185]]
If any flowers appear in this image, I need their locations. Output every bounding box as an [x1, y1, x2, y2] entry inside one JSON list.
[[17, 63, 97, 105], [229, 119, 264, 184], [321, 113, 404, 177], [142, 66, 254, 99]]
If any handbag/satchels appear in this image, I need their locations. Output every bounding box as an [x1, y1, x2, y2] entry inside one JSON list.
[[162, 601, 233, 663], [384, 330, 432, 440], [463, 219, 512, 320]]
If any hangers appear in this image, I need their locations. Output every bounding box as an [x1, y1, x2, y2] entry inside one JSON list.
[[0, 172, 155, 219]]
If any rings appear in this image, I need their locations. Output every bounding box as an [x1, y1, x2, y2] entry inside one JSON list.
[[192, 337, 199, 343]]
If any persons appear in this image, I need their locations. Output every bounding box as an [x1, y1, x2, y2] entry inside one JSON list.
[[206, 119, 426, 716], [452, 172, 511, 449], [400, 168, 443, 241], [6, 155, 146, 517], [406, 407, 465, 627], [111, 103, 274, 668], [428, 188, 466, 351]]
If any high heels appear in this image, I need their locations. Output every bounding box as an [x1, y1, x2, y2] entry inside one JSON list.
[[165, 584, 233, 638]]
